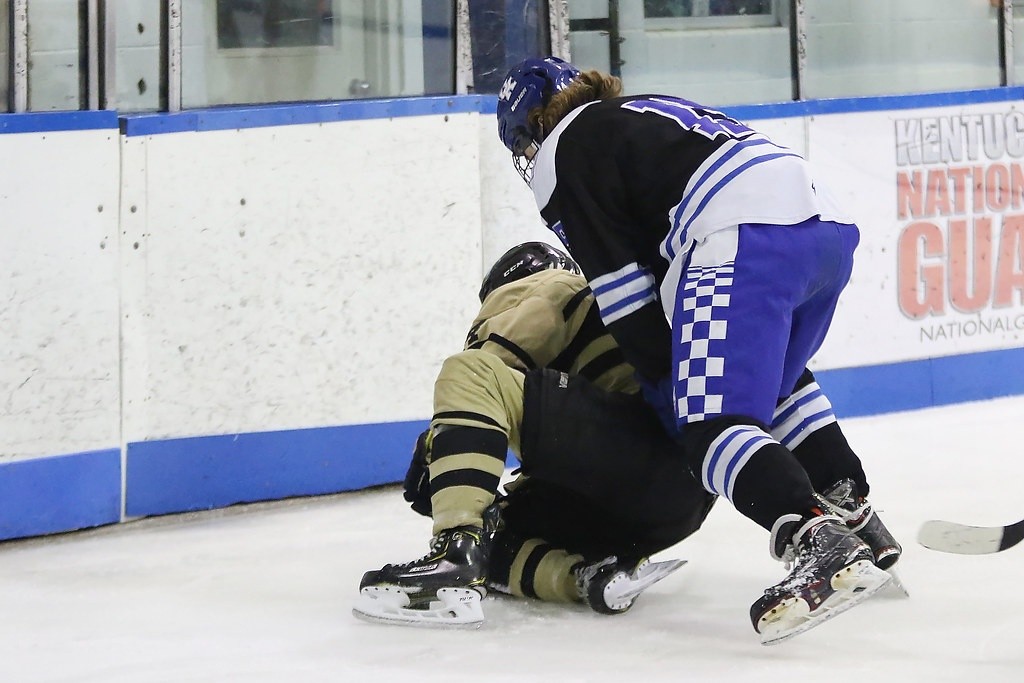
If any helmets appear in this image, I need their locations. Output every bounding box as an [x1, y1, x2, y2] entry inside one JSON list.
[[497, 55, 582, 190], [478, 241, 581, 304]]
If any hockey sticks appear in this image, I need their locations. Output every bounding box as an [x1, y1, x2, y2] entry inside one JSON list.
[[917, 518, 1023, 553]]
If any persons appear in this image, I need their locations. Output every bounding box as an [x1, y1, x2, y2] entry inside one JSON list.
[[497, 57, 910, 645], [352, 241, 719, 626]]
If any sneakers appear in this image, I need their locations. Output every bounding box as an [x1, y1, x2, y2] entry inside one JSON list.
[[750, 513, 894, 646], [351, 524, 485, 631], [817, 477, 902, 571], [568, 555, 687, 615]]
